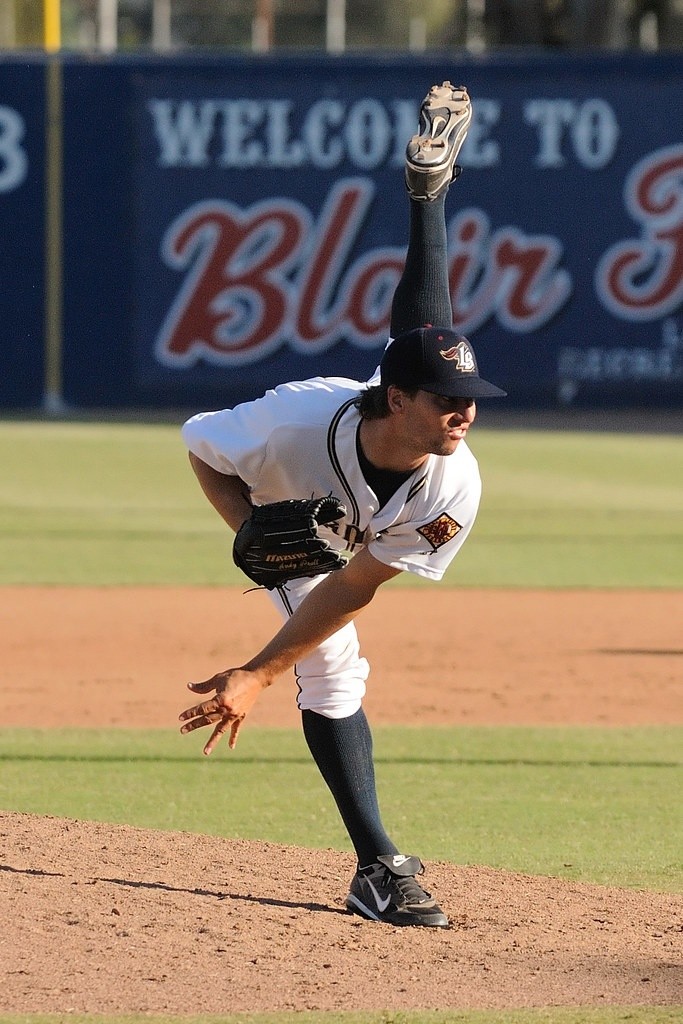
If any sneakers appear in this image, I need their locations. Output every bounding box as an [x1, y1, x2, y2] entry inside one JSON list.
[[403, 80, 474, 203], [345, 854, 449, 927]]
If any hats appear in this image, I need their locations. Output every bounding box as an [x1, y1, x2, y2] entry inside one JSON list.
[[379, 322, 509, 398]]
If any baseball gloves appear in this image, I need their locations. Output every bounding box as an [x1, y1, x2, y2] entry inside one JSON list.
[[229, 492, 354, 595]]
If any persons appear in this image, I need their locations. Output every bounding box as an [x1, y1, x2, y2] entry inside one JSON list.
[[178, 80, 483, 927]]
